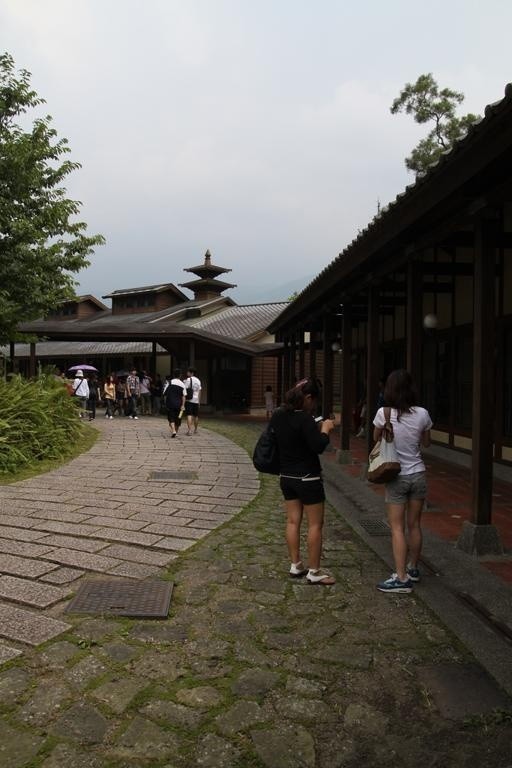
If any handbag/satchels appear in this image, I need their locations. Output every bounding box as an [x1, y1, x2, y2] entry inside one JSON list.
[[366, 406, 402, 484], [185, 378, 194, 399], [252, 406, 300, 474]]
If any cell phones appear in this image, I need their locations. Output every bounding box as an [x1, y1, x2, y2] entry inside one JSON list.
[[314, 416, 324, 424]]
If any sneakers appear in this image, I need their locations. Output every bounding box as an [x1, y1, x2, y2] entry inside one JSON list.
[[288, 559, 308, 577], [170, 431, 177, 438], [306, 569, 336, 585], [377, 575, 412, 594], [185, 430, 192, 435], [109, 416, 114, 419], [134, 416, 139, 420], [105, 415, 109, 419], [407, 568, 419, 581], [128, 415, 133, 419]]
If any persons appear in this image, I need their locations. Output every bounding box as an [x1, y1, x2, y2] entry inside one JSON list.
[[371, 371, 434, 595], [266, 375, 337, 586], [183, 366, 202, 436], [353, 396, 368, 440], [57, 361, 170, 419], [163, 368, 188, 437], [264, 385, 274, 420]]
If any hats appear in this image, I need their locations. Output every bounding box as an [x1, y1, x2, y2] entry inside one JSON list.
[[75, 369, 84, 376]]
